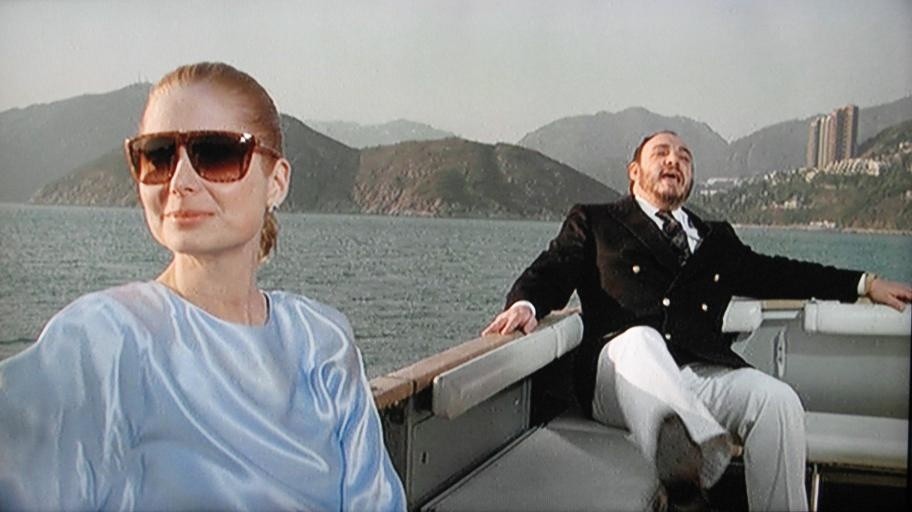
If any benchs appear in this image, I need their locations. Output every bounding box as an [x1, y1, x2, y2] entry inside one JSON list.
[[363, 294, 911, 512]]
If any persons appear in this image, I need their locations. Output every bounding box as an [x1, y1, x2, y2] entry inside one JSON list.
[[482, 130, 912, 512], [0, 63, 407, 512]]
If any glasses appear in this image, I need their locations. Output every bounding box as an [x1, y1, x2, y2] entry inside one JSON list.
[[125, 131, 284, 185]]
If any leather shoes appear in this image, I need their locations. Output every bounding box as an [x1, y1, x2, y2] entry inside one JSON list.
[[654, 414, 704, 511]]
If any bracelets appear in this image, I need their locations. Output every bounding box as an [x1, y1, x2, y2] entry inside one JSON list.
[[864, 269, 877, 297]]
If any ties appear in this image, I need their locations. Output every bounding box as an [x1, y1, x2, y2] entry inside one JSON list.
[[654, 208, 693, 263]]
